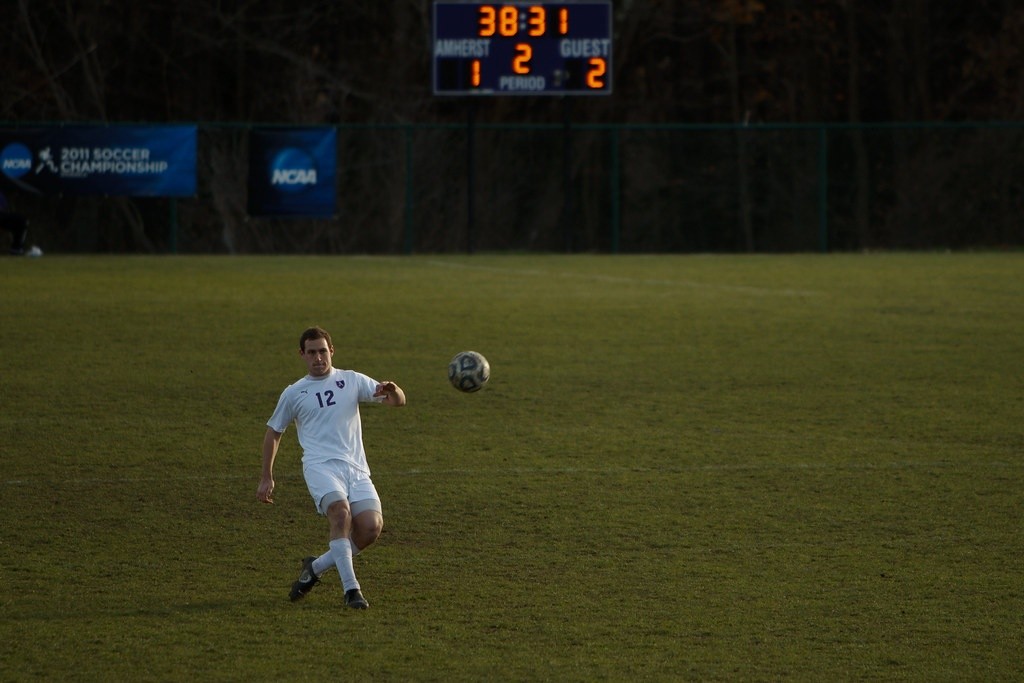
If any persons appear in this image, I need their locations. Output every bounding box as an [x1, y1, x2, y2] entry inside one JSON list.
[[258, 327, 407, 611]]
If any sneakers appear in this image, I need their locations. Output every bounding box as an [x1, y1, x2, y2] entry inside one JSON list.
[[289, 555, 323, 602], [344, 589, 369, 610]]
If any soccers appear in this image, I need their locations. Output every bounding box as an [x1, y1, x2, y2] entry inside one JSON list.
[[447, 350, 491, 394]]
